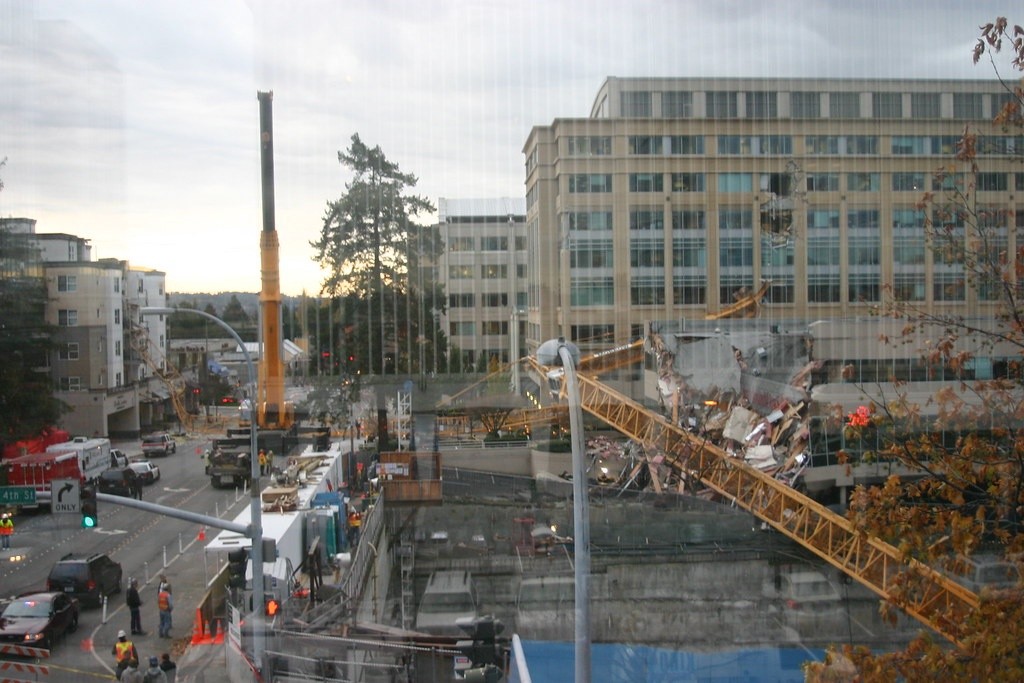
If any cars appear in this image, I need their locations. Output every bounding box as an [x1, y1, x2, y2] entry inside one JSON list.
[[0, 591, 80, 653], [110, 448, 128, 469], [129, 459, 161, 484], [784, 570, 849, 635]]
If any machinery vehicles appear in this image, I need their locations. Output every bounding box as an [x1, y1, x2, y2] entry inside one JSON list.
[[203, 93, 303, 489]]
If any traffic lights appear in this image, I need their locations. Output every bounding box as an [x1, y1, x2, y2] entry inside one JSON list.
[[228, 547, 246, 590], [221, 397, 236, 404], [455, 614, 506, 683], [192, 389, 201, 394], [265, 601, 280, 619], [80, 484, 98, 529]]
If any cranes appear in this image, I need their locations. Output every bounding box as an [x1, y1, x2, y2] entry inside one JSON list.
[[534, 267, 985, 652]]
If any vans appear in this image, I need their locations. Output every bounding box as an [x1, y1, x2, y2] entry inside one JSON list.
[[430, 531, 489, 556], [415, 568, 478, 645]]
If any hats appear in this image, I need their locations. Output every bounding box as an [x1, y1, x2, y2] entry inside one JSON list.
[[150, 655, 158, 664]]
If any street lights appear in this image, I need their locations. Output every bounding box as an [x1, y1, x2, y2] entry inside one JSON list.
[[139, 306, 267, 675], [535, 336, 592, 683]]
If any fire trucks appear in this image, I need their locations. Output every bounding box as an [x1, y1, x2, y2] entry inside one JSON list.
[[2, 436, 111, 512]]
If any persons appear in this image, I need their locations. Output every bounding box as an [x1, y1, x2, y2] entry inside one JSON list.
[[347, 507, 361, 548], [120, 657, 144, 683], [159, 653, 176, 683], [111, 630, 139, 667], [115, 650, 131, 680], [158, 583, 174, 639], [133, 473, 143, 500], [158, 575, 173, 628], [204, 450, 212, 475], [143, 656, 168, 683], [0, 514, 14, 551], [125, 578, 145, 635], [266, 450, 274, 473], [259, 449, 267, 476]]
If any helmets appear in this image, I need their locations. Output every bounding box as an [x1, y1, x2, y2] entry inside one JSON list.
[[117, 630, 126, 638], [3, 514, 8, 518], [163, 582, 169, 588]]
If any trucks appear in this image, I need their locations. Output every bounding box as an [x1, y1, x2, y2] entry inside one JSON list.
[[509, 541, 574, 641]]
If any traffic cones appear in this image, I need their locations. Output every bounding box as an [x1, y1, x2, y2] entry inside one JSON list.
[[190, 619, 202, 645], [212, 619, 224, 644], [196, 527, 206, 541], [201, 619, 214, 644]]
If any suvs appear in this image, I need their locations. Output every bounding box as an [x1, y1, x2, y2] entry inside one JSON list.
[[142, 432, 177, 457], [940, 552, 1020, 597], [47, 551, 123, 608], [97, 467, 141, 497]]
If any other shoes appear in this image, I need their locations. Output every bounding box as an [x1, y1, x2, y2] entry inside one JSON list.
[[2, 548, 6, 550], [6, 548, 10, 551]]
